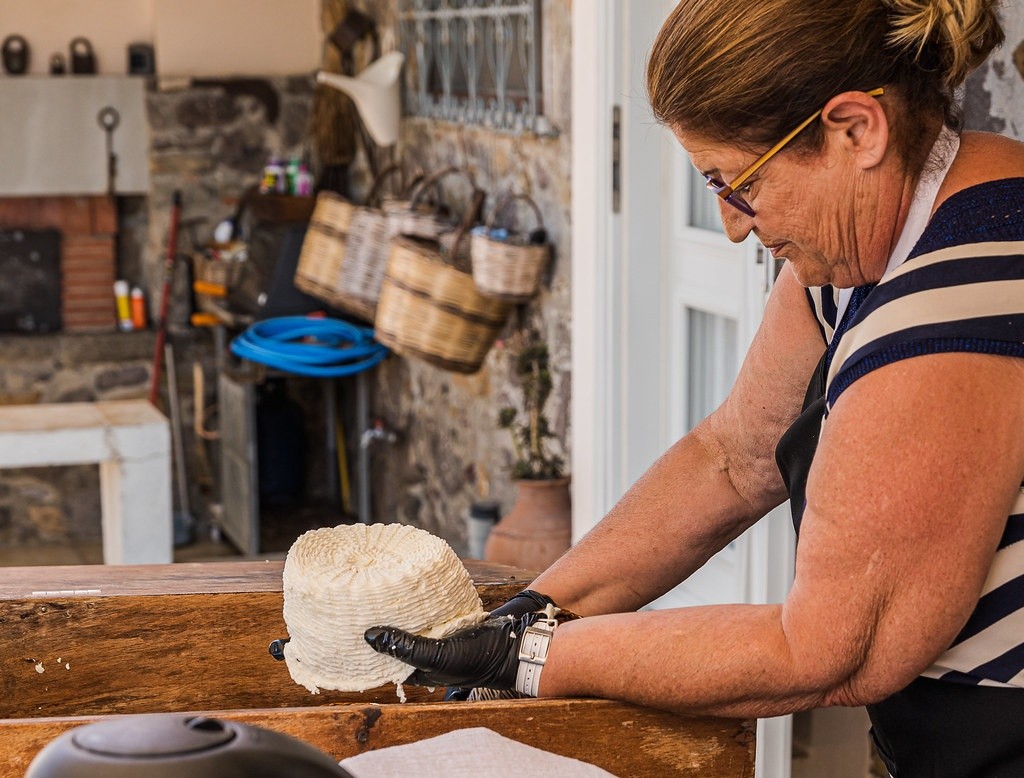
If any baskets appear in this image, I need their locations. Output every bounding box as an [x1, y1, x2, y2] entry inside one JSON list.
[[294, 162, 556, 379]]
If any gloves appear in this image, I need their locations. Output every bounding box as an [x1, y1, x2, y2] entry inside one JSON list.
[[266, 589, 575, 710]]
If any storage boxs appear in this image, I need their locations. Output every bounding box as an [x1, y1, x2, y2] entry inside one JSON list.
[[290, 162, 555, 373]]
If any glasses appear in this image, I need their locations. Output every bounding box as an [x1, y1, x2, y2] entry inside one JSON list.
[[706, 87, 888, 219]]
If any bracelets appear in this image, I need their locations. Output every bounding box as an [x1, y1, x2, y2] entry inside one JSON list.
[[515, 622, 555, 697]]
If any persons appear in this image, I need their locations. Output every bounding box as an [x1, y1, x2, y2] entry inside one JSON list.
[[266, 0, 1024, 778]]
